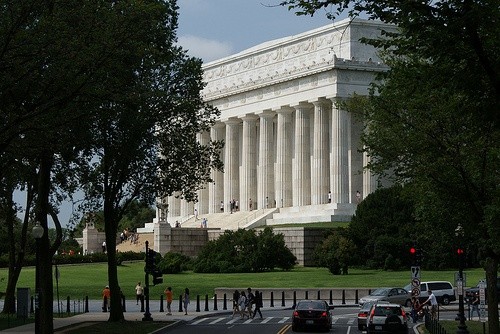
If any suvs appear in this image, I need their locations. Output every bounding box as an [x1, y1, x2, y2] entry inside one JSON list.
[[464, 279, 488, 305], [404, 280, 457, 306], [364, 303, 409, 334]]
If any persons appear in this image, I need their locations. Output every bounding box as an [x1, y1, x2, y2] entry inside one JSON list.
[[102, 241, 106, 253], [249, 198, 253, 211], [266, 197, 269, 208], [175, 220, 181, 228], [233, 288, 263, 320], [183, 288, 190, 315], [228, 199, 239, 214], [418, 290, 438, 320], [194, 209, 199, 222], [101, 285, 111, 312], [201, 218, 208, 229], [356, 191, 361, 202], [62, 249, 75, 256], [327, 191, 332, 203], [164, 286, 174, 315], [135, 282, 143, 305], [120, 227, 139, 245], [468, 291, 481, 321], [220, 200, 224, 211]]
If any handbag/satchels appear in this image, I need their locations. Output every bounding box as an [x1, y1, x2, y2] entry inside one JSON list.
[[244, 297, 249, 306]]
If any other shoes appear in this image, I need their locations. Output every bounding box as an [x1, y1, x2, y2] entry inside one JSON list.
[[166, 313, 172, 315], [259, 317, 264, 320], [249, 316, 253, 319], [240, 318, 244, 320], [247, 314, 249, 319]]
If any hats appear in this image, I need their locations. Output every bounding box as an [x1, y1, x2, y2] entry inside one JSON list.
[[105, 285, 109, 288]]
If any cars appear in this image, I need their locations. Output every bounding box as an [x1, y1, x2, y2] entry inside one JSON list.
[[291, 299, 335, 333], [358, 287, 412, 309], [358, 300, 390, 331]]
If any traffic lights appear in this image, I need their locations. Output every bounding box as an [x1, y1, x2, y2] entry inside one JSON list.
[[457, 247, 463, 260], [411, 248, 421, 267]]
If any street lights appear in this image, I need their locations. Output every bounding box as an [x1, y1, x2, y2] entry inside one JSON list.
[[32, 220, 44, 334]]
